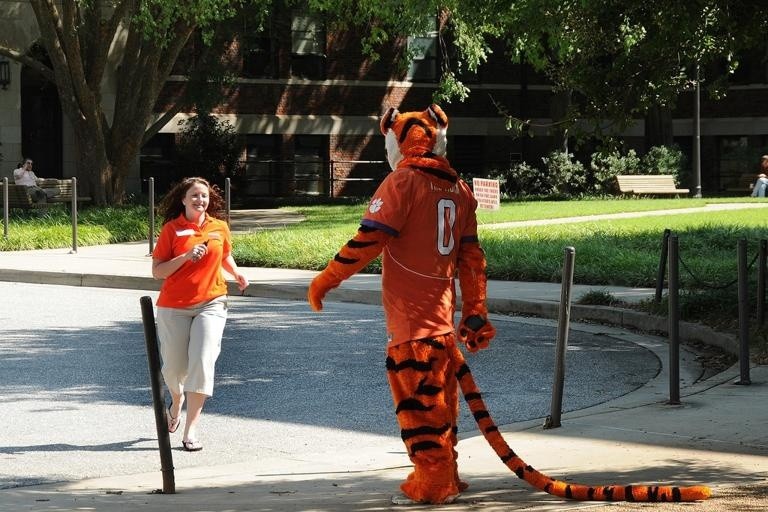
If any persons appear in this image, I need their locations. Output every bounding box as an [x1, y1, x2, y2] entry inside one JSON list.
[[150, 175, 250, 451], [12, 158, 62, 209], [748, 154, 768, 197]]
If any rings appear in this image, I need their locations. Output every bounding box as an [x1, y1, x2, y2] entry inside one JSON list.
[[197, 249, 200, 254]]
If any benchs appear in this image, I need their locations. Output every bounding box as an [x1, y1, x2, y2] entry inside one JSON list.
[[0, 179, 91, 214], [613, 174, 690, 199], [725, 173, 768, 197]]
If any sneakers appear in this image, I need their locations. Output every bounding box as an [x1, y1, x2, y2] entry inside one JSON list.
[[390, 489, 461, 505]]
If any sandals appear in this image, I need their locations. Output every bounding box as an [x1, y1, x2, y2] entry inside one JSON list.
[[166, 400, 181, 434], [182, 439, 203, 452]]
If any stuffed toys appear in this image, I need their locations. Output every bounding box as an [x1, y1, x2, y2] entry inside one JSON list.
[[307, 101, 713, 506]]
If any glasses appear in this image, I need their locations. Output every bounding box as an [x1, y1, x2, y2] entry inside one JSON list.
[[27, 162, 33, 165]]
